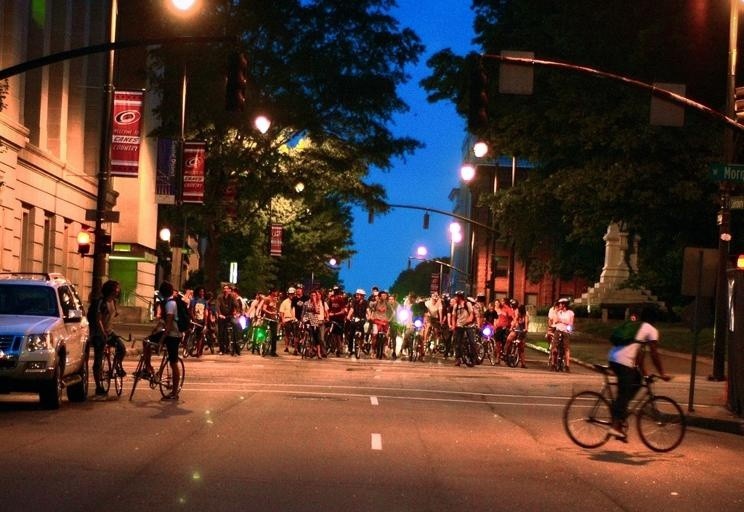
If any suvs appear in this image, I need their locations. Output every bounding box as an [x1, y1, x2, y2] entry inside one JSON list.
[[0, 271, 91, 411]]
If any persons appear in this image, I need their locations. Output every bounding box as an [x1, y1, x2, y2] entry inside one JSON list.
[[90, 280, 127, 395], [603, 308, 675, 444], [135, 280, 530, 401], [545, 302, 560, 343], [551, 298, 575, 372]]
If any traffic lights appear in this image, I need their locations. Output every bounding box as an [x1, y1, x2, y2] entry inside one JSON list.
[[732, 86, 744, 121], [727, 197, 744, 210], [222, 46, 248, 112], [160, 228, 170, 251], [98, 229, 111, 255], [76, 230, 88, 253]]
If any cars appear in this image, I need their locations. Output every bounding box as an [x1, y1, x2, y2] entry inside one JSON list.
[[152, 291, 182, 304]]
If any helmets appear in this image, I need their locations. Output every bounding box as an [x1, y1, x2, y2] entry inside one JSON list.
[[356, 289, 366, 295], [455, 290, 464, 296], [558, 298, 570, 306], [287, 287, 296, 295]]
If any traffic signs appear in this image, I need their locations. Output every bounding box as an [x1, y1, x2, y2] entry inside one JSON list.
[[706, 162, 744, 181]]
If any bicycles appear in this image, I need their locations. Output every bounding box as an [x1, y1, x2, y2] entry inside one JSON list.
[[94, 334, 131, 395], [556, 362, 688, 455], [127, 335, 184, 399], [544, 326, 571, 371]]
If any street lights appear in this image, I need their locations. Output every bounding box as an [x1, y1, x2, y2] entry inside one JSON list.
[[90, 0, 201, 346], [471, 140, 514, 307], [462, 156, 498, 300]]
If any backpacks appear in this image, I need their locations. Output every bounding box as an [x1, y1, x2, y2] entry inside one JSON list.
[[165, 297, 190, 332], [609, 319, 647, 346]]
